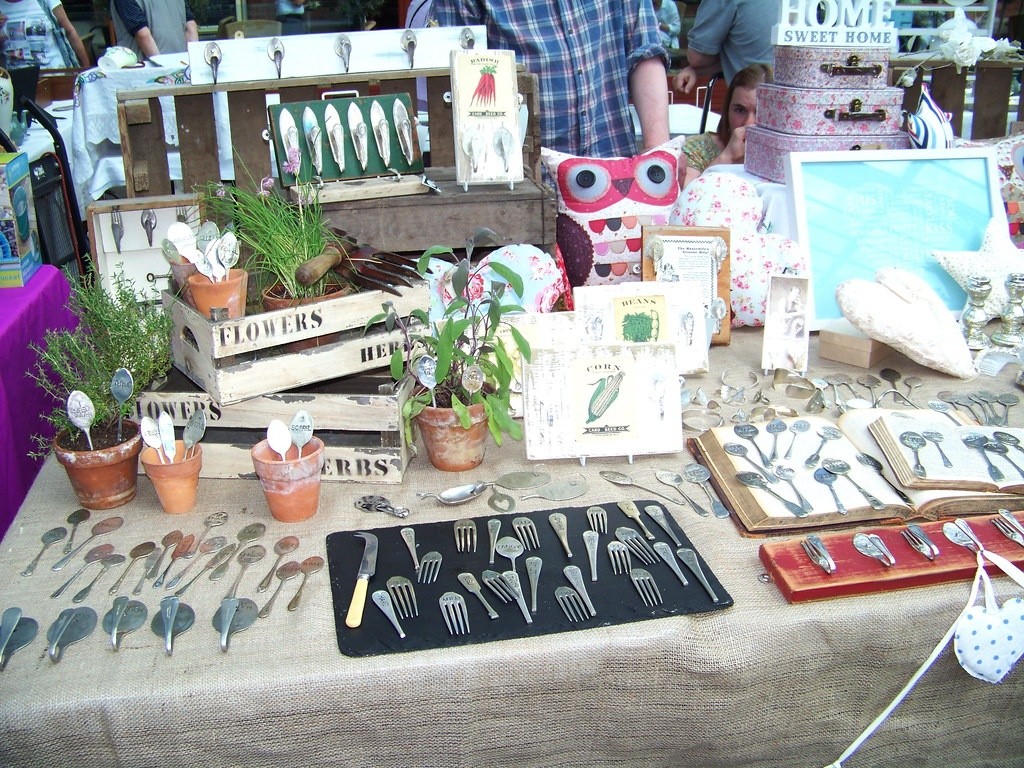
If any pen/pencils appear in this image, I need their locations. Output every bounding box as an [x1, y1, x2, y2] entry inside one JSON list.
[[56, 117, 67, 119]]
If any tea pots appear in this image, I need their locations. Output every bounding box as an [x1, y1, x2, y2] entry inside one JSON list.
[[10, 110, 28, 146]]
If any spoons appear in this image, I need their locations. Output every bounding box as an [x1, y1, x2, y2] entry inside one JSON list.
[[141, 209, 157, 247], [853, 533, 896, 567], [267, 410, 313, 461], [599, 368, 1024, 517], [942, 518, 986, 554], [20, 510, 325, 620], [140, 409, 206, 464], [162, 221, 239, 284]]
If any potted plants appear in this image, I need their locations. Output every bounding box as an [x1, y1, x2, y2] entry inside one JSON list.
[[361, 227, 532, 472], [24, 251, 175, 511], [194, 184, 351, 355]]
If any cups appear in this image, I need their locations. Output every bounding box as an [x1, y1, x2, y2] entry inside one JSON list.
[[98, 46, 138, 70]]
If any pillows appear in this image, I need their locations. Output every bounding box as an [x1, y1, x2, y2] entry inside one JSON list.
[[539, 134, 686, 312]]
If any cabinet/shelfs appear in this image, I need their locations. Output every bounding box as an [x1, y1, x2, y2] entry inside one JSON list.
[[893, 0, 997, 50]]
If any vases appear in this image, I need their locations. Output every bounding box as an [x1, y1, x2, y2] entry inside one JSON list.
[[193, 269, 248, 321], [252, 435, 325, 524], [167, 260, 203, 310], [141, 439, 202, 514]]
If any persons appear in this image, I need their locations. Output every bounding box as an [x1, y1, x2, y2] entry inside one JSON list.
[[0, 0, 91, 76], [273, 0, 783, 190], [110, 0, 198, 60]]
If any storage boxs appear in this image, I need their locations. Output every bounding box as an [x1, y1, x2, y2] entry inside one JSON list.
[[819, 317, 897, 369], [0, 153, 43, 289]]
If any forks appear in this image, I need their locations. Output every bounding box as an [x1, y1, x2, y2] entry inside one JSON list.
[[800, 534, 836, 574], [176, 206, 188, 225], [990, 508, 1024, 547], [901, 524, 940, 561], [110, 212, 125, 254], [382, 506, 665, 637]]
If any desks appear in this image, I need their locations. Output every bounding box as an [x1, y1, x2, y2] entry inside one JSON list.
[[73, 52, 216, 220], [0, 328, 1023, 768]]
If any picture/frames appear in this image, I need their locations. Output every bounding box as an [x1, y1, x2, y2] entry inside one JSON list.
[[783, 145, 1001, 332]]
